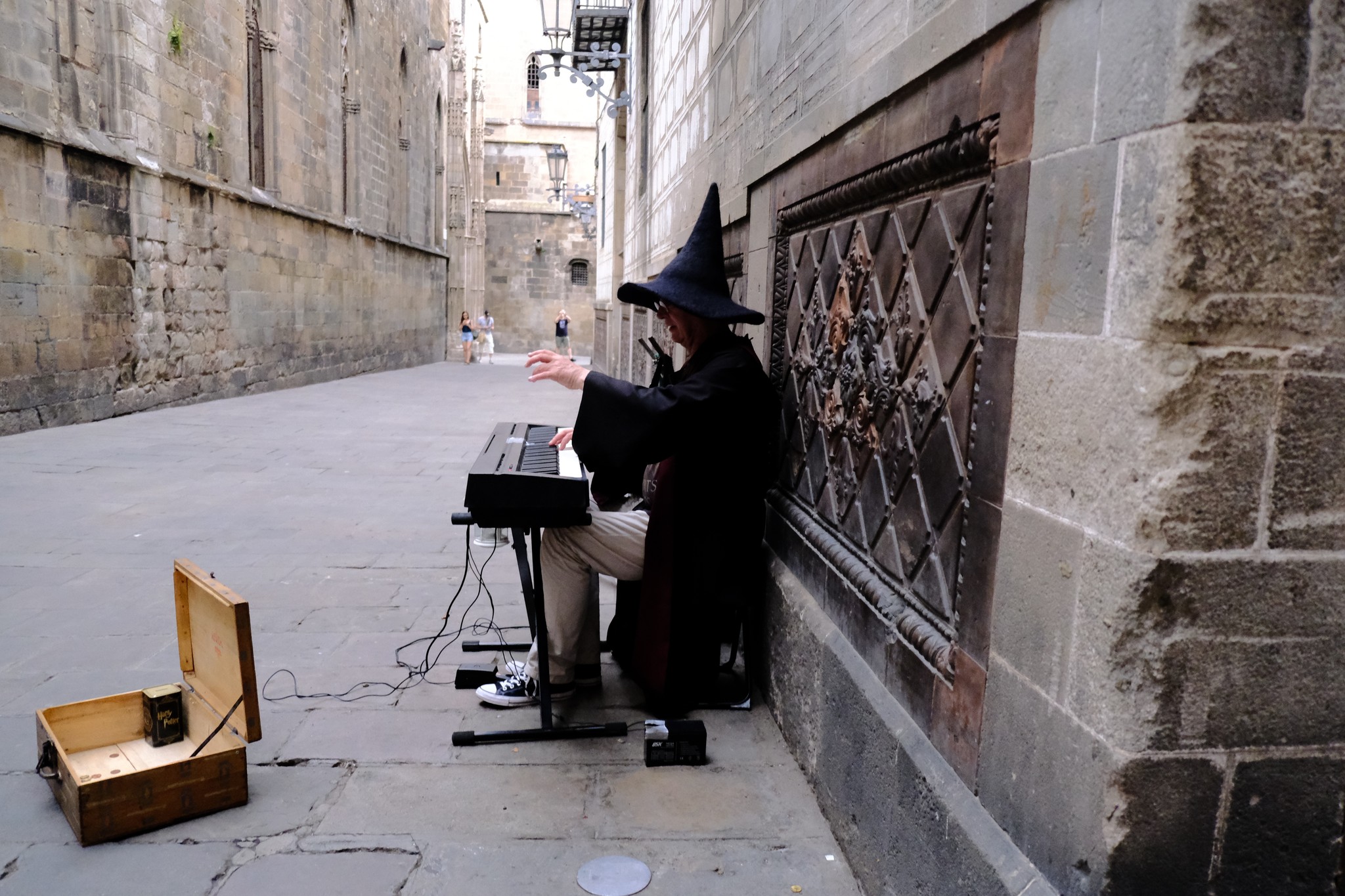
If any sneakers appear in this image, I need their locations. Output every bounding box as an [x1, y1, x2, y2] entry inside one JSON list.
[[475, 671, 574, 706]]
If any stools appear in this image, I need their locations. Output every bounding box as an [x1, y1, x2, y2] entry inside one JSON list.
[[716, 609, 759, 709]]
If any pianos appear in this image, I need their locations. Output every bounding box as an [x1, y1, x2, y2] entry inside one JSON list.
[[451, 420, 627, 749]]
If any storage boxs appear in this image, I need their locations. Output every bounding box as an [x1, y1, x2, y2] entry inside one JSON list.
[[33, 555, 265, 850], [142, 684, 184, 747]]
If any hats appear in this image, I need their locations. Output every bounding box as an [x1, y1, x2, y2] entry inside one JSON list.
[[617, 182, 765, 325]]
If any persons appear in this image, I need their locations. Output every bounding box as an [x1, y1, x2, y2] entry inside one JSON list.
[[555, 310, 577, 363], [475, 182, 783, 707], [475, 310, 495, 364], [457, 312, 475, 364]]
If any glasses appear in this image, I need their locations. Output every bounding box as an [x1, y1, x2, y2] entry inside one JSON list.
[[654, 298, 674, 313]]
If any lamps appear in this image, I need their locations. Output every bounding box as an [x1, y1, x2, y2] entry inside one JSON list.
[[546, 144, 597, 242], [533, 0, 632, 119]]
